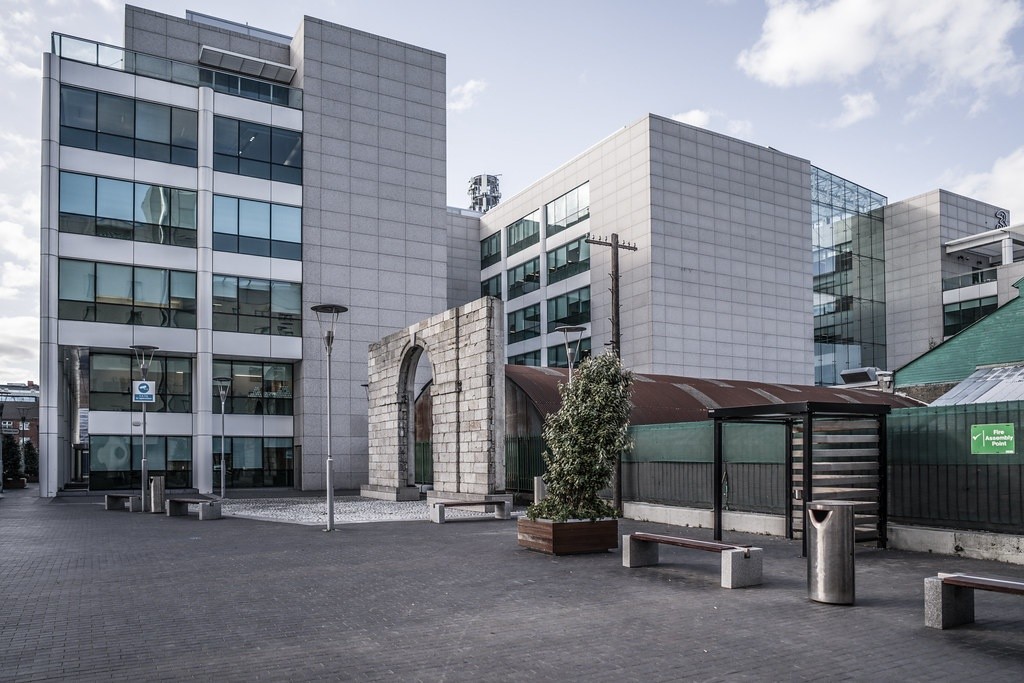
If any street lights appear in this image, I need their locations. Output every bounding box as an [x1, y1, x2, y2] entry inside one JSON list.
[[128, 344, 159, 513], [311, 303, 348, 531], [16, 405, 31, 490], [213, 376, 235, 498], [554, 324, 587, 383]]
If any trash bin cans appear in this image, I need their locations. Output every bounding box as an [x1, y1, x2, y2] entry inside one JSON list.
[[804, 501, 858, 605], [148, 474, 166, 514]]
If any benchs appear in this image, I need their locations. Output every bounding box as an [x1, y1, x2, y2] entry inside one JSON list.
[[165, 498, 220, 521], [106, 493, 140, 513], [623, 530, 763, 589], [423, 500, 510, 524], [925, 572, 1024, 629]]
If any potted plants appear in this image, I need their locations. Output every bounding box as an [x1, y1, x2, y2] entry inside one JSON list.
[[23, 441, 39, 482], [2, 433, 27, 488], [517, 351, 650, 554]]
